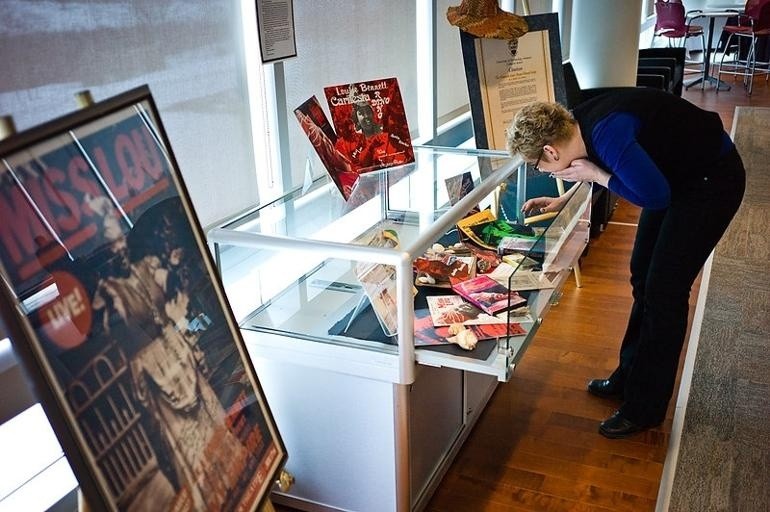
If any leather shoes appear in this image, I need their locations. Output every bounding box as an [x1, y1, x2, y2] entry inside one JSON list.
[[599, 406, 664, 439], [587, 379, 625, 397]]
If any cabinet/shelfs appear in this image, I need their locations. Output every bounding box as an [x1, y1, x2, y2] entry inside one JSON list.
[[206, 145, 527, 510]]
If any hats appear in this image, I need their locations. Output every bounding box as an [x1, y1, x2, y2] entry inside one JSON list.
[[446, 0, 529, 40]]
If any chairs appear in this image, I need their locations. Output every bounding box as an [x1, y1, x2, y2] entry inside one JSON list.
[[716, 0, 770, 97], [651, 0, 707, 91], [636, 47, 686, 100]]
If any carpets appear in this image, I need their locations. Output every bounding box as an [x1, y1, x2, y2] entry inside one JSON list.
[[655, 105, 770, 511]]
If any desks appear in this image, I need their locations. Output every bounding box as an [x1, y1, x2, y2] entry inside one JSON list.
[[683, 11, 739, 93]]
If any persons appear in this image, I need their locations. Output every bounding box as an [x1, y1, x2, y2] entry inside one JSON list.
[[344, 94, 409, 167], [78, 195, 258, 510], [503, 84, 746, 441]]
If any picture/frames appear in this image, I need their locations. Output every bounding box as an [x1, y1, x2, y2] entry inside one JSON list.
[[1, 85, 289, 511]]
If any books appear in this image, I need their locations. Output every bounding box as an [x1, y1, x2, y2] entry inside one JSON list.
[[345, 167, 579, 347]]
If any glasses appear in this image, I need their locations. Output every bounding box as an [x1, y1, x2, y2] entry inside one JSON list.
[[528, 149, 545, 173]]
